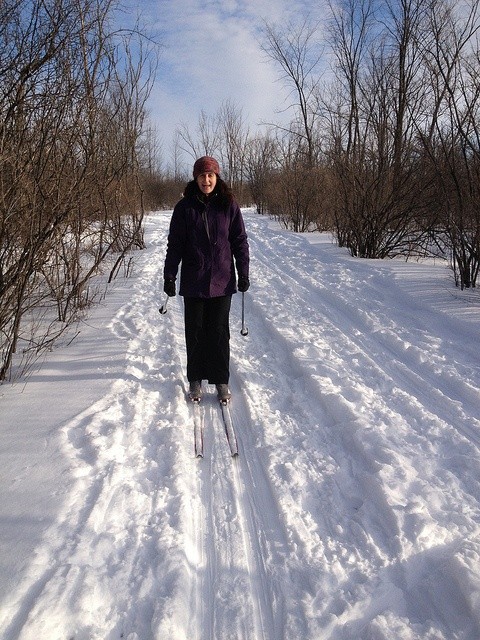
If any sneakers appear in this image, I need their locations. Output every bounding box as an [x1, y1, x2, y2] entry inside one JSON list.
[[190, 380, 202, 401], [216, 383, 230, 402]]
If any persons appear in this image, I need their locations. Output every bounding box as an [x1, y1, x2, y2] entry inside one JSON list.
[[164, 156, 249, 402]]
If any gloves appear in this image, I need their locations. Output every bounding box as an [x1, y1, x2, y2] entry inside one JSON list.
[[163, 276, 177, 297], [237, 276, 250, 292]]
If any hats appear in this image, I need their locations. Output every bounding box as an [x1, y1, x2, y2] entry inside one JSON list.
[[193, 156, 220, 179]]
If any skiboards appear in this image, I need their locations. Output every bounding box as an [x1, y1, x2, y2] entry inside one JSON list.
[[193, 401, 239, 458]]
